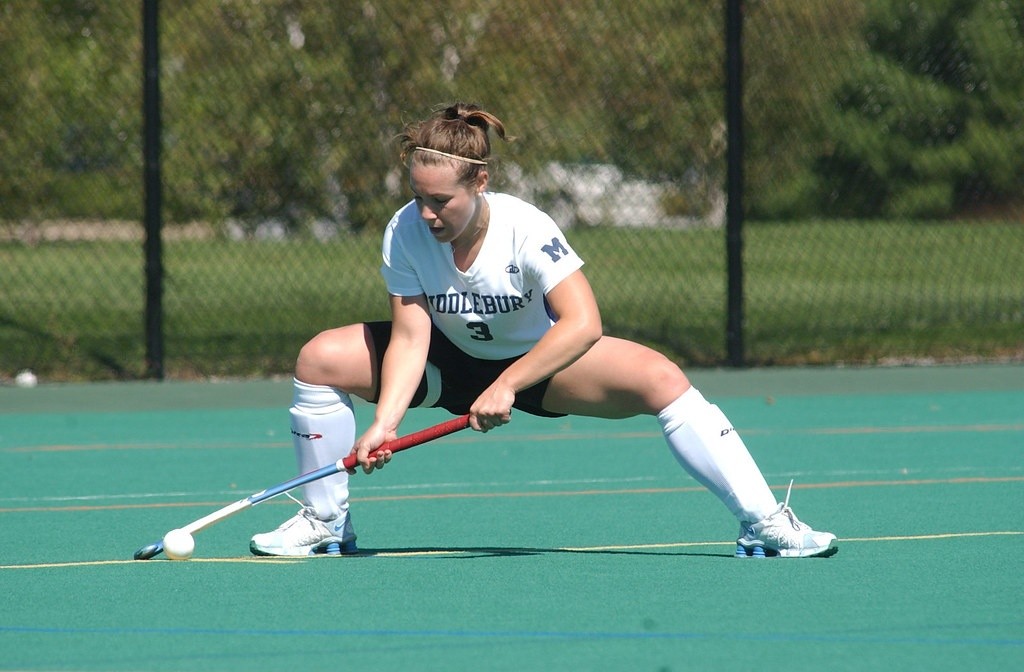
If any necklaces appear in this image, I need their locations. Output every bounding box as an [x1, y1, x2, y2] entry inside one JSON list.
[[444, 206, 489, 255]]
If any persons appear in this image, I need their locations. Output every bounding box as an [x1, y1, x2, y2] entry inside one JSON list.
[[249, 100, 839, 559]]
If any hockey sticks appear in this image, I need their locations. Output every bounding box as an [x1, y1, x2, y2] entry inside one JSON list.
[[132, 409, 510, 561]]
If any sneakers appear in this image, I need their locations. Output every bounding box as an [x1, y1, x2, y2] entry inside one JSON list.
[[248, 490, 360, 557], [734, 479, 840, 560]]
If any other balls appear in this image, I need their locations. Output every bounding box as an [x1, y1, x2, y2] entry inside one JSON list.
[[163, 528, 194, 561], [15, 372, 38, 388]]
